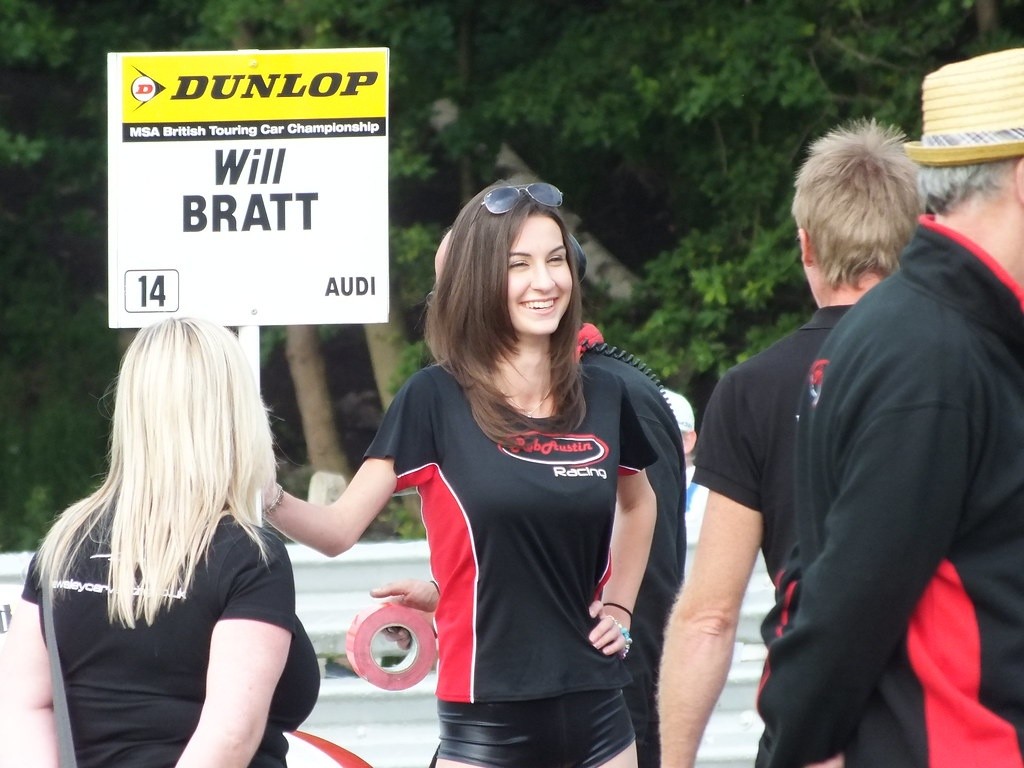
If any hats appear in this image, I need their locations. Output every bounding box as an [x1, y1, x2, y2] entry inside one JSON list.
[[905, 46, 1024, 167]]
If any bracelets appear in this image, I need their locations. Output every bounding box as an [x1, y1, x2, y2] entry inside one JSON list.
[[431, 580, 440, 595], [262, 483, 284, 515], [603, 602, 633, 616], [608, 615, 632, 660]]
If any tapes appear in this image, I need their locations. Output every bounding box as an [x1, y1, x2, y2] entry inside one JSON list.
[[345, 603, 437, 691]]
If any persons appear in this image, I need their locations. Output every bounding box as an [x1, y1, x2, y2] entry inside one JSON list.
[[0, 317, 320, 768], [655, 385, 709, 581], [757, 46, 1024, 768], [259, 177, 659, 768], [657, 120, 921, 768], [370, 214, 687, 768]]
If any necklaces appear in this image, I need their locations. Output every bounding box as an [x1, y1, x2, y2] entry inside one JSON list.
[[496, 382, 553, 418]]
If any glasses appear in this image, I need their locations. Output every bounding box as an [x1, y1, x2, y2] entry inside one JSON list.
[[472, 182, 563, 229]]
